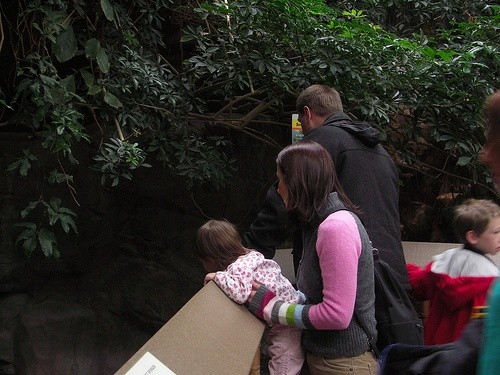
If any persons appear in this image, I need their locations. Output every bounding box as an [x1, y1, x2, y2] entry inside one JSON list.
[[398, 196, 500, 352], [195, 217, 309, 375], [243, 141, 384, 375], [240, 84, 425, 375], [476, 84, 500, 375]]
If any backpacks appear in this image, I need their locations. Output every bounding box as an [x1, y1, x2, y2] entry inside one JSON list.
[[318, 205, 424, 363]]
